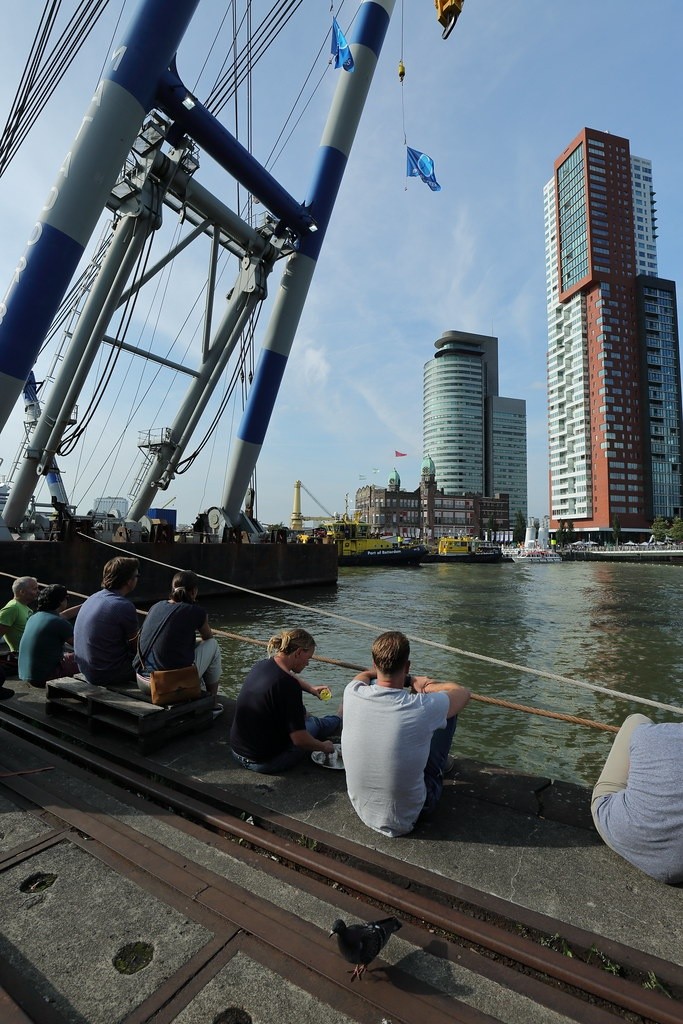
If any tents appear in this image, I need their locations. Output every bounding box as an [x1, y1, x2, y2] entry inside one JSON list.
[[571, 541, 599, 545], [624, 540, 664, 546]]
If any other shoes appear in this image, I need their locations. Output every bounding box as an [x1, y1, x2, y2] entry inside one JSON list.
[[441, 755, 454, 776]]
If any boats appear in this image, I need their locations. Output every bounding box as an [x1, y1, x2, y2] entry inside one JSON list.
[[510, 548, 563, 564], [420, 533, 503, 564], [294, 492, 429, 567]]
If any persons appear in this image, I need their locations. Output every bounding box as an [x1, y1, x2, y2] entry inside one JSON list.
[[73, 556, 141, 686], [506, 542, 585, 551], [132, 570, 224, 720], [591, 713, 683, 884], [341, 631, 471, 838], [229, 628, 343, 775], [292, 531, 321, 544], [0, 576, 79, 688]]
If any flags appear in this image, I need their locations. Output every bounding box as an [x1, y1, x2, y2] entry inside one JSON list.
[[405, 146, 442, 192], [331, 16, 356, 72], [396, 451, 407, 457]]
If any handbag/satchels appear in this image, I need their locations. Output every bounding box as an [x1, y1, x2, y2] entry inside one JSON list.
[[150, 666, 202, 705]]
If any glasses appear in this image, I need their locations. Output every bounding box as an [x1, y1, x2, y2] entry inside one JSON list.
[[130, 573, 141, 579]]
